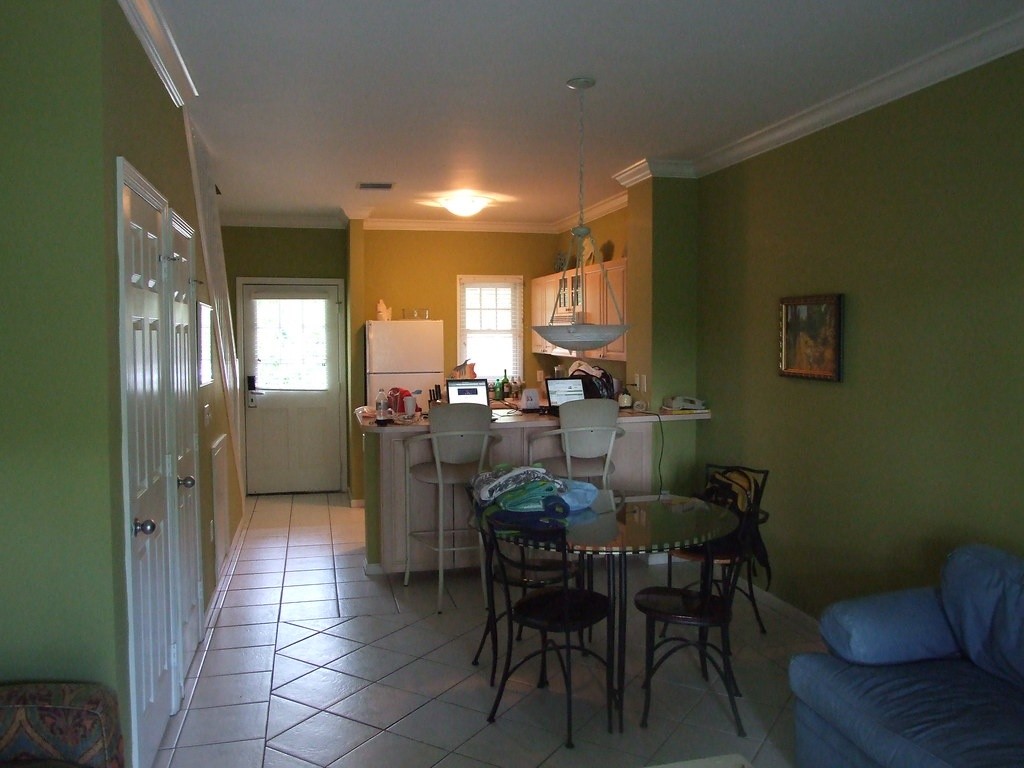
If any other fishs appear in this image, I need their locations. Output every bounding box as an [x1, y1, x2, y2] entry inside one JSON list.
[[451, 358, 476, 379]]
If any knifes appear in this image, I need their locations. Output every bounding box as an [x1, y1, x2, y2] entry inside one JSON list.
[[429, 384, 442, 401]]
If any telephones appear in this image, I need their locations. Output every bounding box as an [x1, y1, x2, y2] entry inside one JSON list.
[[663, 397, 706, 410]]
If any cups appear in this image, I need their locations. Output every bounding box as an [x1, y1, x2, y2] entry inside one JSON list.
[[554, 366, 565, 378], [403, 396, 416, 416]]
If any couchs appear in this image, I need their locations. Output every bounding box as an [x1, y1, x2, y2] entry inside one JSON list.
[[788, 541, 1023, 768]]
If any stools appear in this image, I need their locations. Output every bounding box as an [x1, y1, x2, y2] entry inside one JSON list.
[[529, 399, 625, 587], [403, 403, 490, 615]]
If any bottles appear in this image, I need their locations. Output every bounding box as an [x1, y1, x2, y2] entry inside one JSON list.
[[376, 388, 388, 420], [517, 377, 522, 400], [513, 382, 519, 402], [495, 379, 502, 400], [488, 380, 495, 401], [501, 369, 510, 398], [511, 376, 514, 384]]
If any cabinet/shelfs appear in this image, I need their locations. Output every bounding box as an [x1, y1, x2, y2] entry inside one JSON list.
[[531, 258, 627, 361]]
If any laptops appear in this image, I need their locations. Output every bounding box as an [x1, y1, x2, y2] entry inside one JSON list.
[[446, 379, 498, 421], [545, 377, 587, 417]]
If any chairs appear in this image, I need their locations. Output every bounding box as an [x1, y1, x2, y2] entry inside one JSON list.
[[471, 484, 588, 665], [633, 504, 753, 738], [659, 463, 770, 656], [485, 515, 619, 748]]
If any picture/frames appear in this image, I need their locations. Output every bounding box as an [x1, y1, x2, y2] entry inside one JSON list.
[[778, 294, 846, 382]]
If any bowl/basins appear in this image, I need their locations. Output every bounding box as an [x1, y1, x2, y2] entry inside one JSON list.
[[505, 398, 513, 402]]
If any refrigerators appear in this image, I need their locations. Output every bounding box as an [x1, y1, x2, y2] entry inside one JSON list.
[[364, 320, 446, 411]]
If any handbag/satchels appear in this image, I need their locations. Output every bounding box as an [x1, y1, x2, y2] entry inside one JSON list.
[[568, 366, 614, 399]]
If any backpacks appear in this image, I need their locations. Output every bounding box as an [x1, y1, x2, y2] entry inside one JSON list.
[[678, 467, 772, 591]]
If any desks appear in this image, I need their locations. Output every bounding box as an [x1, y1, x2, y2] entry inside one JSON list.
[[468, 489, 739, 733]]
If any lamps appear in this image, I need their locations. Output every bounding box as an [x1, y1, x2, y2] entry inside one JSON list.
[[532, 77, 628, 352]]
